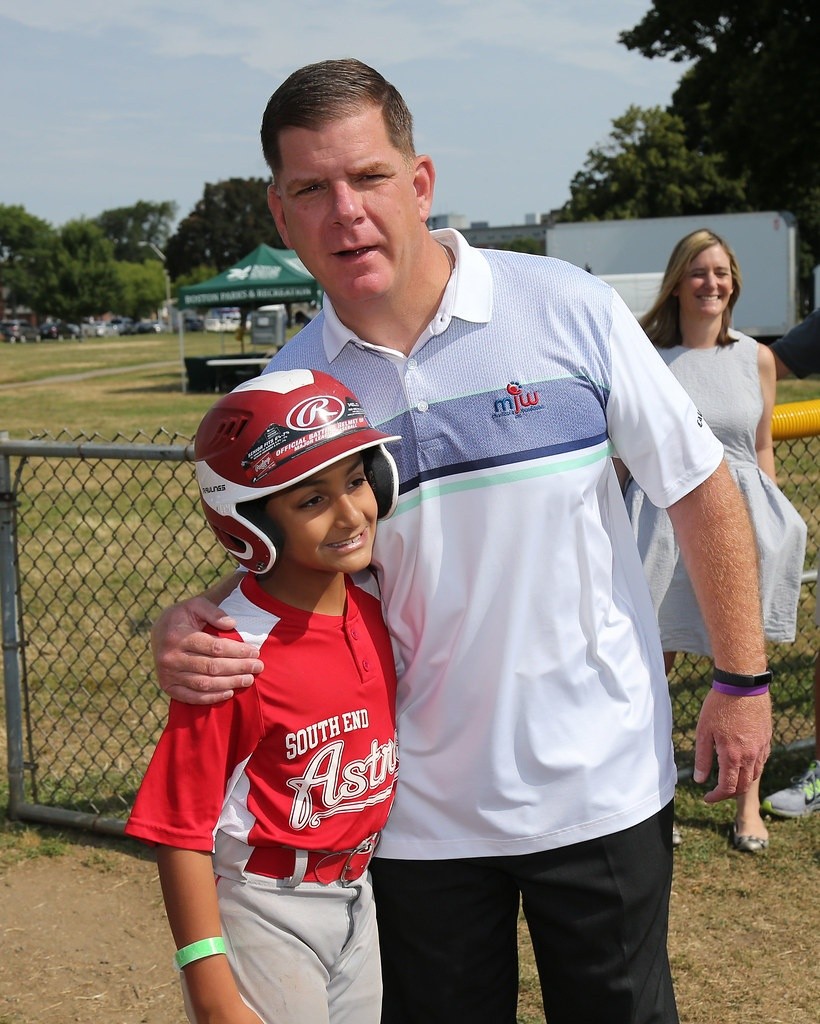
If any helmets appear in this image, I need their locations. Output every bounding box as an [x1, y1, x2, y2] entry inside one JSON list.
[[194, 368, 402, 575]]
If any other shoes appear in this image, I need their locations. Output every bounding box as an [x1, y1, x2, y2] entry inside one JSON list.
[[733, 810, 769, 852], [673, 825, 682, 846]]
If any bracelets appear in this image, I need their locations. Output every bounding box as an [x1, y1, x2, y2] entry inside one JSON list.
[[712, 667, 772, 698], [174, 937, 227, 970]]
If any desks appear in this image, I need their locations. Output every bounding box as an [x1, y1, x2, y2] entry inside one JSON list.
[[205, 359, 271, 392]]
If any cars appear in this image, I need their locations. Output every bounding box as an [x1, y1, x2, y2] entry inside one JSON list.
[[0, 319, 38, 344], [66, 315, 203, 338], [39, 322, 78, 340]]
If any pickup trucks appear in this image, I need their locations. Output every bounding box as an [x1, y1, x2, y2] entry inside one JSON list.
[[204, 312, 251, 332]]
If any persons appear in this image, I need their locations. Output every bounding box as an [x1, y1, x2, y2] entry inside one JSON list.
[[610, 227, 805, 851], [761, 309, 820, 819], [260, 58, 772, 1024], [119, 368, 401, 1023]]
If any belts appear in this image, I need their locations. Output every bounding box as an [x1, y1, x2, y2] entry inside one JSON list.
[[244, 831, 384, 888]]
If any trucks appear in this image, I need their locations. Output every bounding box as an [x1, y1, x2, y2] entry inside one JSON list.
[[544, 210, 798, 337]]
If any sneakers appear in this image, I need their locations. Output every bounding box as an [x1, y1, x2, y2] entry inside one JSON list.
[[763, 760, 820, 817]]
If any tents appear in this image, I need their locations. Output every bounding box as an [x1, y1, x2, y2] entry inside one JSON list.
[[176, 241, 319, 397]]
